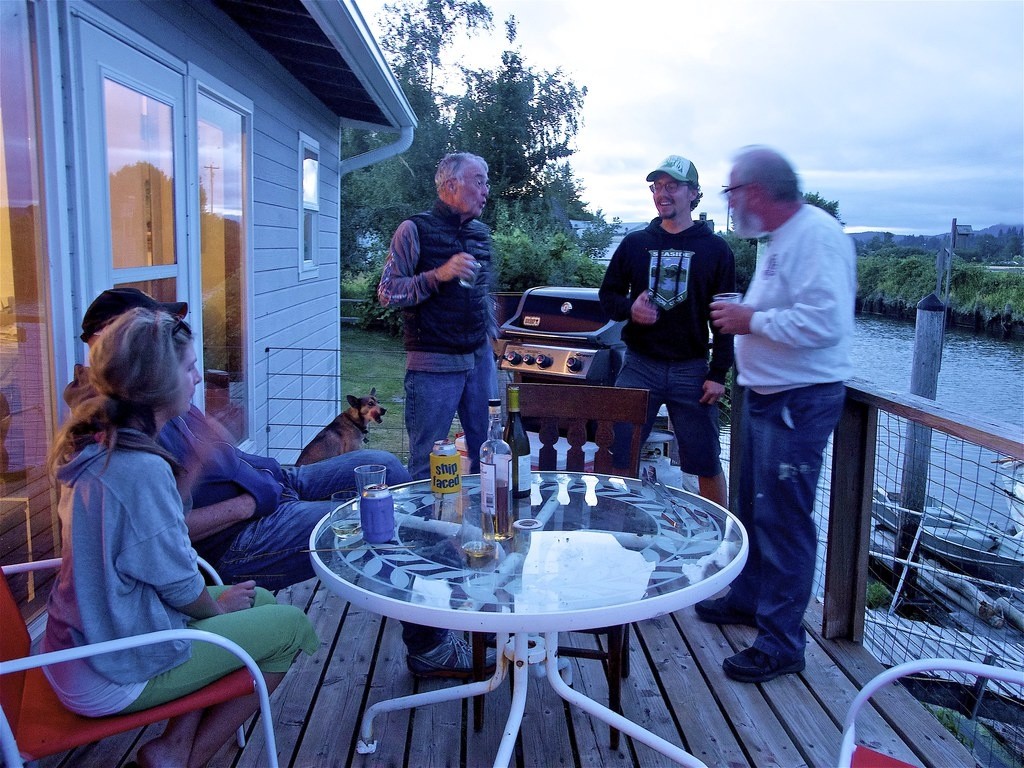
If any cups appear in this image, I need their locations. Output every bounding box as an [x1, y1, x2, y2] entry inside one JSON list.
[[459, 260, 482, 287], [353, 464, 386, 497], [330, 491, 361, 539], [712, 292, 743, 305], [459, 506, 497, 557]]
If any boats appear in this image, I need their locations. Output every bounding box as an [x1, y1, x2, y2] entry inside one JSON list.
[[870, 491, 1024, 594]]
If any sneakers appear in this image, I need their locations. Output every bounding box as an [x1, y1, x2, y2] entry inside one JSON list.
[[723, 648, 805, 684], [695, 600, 758, 627], [406, 629, 497, 681]]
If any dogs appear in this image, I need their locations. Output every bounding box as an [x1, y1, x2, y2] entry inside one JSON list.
[[294, 386, 388, 468]]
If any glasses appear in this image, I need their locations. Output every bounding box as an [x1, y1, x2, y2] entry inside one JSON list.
[[454, 175, 492, 191], [649, 182, 686, 194], [721, 181, 755, 203], [171, 315, 193, 339]]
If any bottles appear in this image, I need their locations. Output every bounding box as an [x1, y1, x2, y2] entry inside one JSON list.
[[503, 386, 532, 499], [480, 399, 514, 542]]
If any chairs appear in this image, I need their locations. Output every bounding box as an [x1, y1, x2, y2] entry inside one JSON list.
[[0, 554, 280, 768], [472, 382, 649, 748]]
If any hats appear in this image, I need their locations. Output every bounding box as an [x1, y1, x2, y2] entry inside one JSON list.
[[80, 287, 188, 342], [646, 156, 699, 187]]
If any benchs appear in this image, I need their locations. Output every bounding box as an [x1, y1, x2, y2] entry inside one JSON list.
[[923, 526, 1000, 551], [892, 505, 953, 528]]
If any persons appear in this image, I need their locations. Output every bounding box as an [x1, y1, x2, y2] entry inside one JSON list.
[[44, 288, 501, 768], [598, 156, 736, 528], [378, 154, 493, 487], [692, 147, 855, 686]]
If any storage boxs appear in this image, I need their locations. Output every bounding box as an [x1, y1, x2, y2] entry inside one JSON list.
[[524, 431, 600, 474]]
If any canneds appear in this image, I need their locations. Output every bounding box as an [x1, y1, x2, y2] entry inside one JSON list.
[[361, 483, 390, 499], [433, 439, 457, 457]]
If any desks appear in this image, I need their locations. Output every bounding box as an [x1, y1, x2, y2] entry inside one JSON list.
[[308, 470, 749, 768]]
